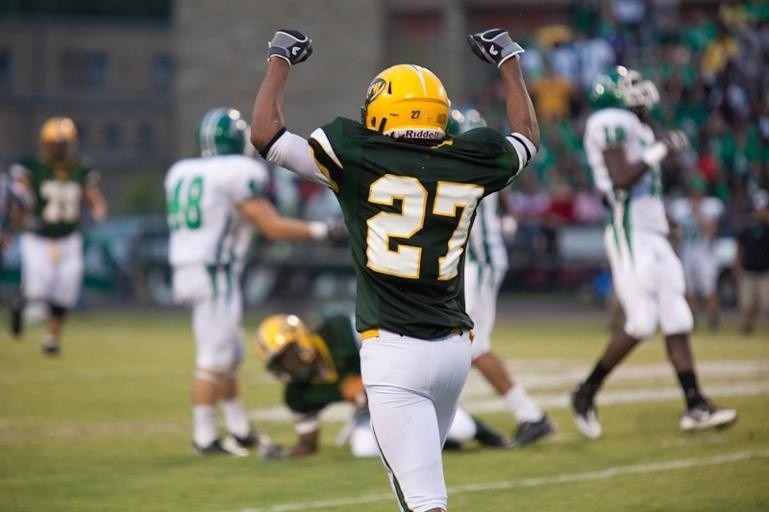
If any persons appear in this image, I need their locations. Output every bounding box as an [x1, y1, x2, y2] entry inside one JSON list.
[[568, 66, 740, 439], [162, 105, 350, 463], [239, 160, 357, 328], [496, 4, 769, 337], [255, 309, 555, 459], [8, 116, 109, 354], [246, 26, 543, 511]]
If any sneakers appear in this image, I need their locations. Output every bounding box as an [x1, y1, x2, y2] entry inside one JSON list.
[[679, 407, 738, 431], [445, 415, 554, 449], [569, 386, 603, 441], [188, 429, 281, 458]]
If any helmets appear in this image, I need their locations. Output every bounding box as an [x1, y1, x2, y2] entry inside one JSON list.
[[199, 107, 254, 158], [258, 313, 319, 368], [39, 118, 77, 169], [590, 67, 661, 108], [361, 65, 449, 143]]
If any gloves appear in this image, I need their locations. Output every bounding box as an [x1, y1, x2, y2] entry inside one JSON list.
[[267, 28, 314, 65], [662, 130, 689, 153], [466, 28, 524, 67]]
